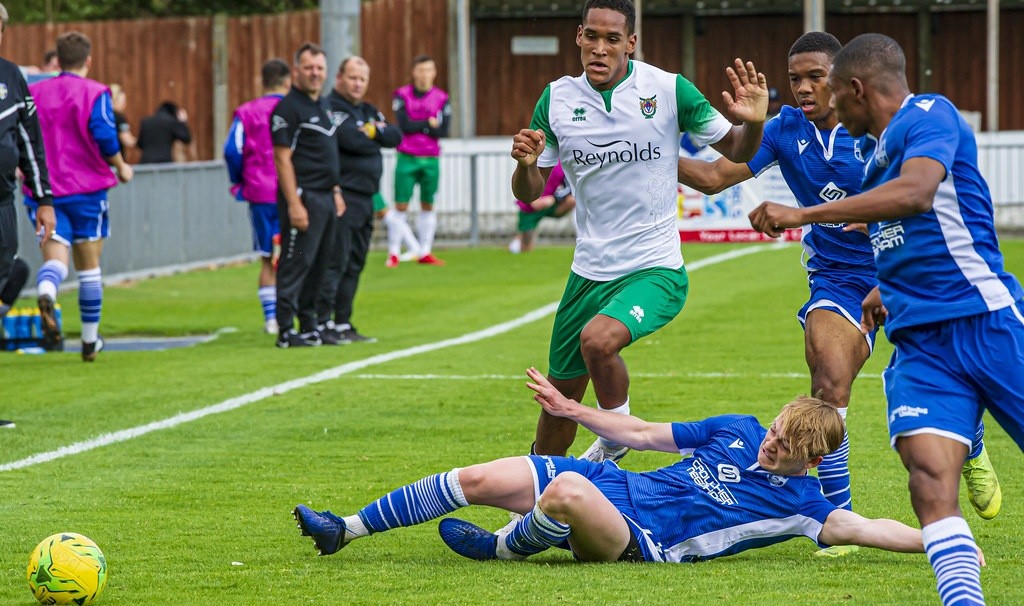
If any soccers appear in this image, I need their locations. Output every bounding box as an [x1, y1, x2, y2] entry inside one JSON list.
[[26, 532, 108, 606]]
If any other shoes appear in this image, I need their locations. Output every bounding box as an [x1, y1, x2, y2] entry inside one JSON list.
[[400, 248, 420, 262], [266, 319, 278, 335], [508, 239, 522, 253]]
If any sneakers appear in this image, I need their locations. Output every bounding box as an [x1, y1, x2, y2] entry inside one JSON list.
[[494, 512, 524, 536], [418, 254, 446, 265], [815, 545, 859, 557], [275, 319, 377, 348], [386, 255, 399, 268], [37, 293, 64, 352], [577, 437, 630, 465], [82, 334, 104, 361], [291, 504, 358, 555], [438, 518, 499, 561], [0, 419, 16, 429], [962, 439, 1002, 520]]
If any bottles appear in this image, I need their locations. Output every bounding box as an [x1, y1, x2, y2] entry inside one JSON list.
[[1, 304, 63, 349]]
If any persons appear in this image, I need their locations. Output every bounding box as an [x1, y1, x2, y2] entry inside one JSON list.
[[750, 33, 1024, 606], [677, 31, 1002, 556], [223, 43, 450, 347], [293, 366, 987, 570], [509, 0, 769, 466], [508, 158, 576, 253], [0, 0, 193, 363]]
